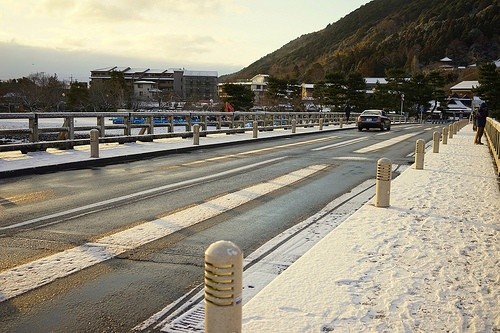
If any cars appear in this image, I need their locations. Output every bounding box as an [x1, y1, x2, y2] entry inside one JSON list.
[[357, 110, 391, 131]]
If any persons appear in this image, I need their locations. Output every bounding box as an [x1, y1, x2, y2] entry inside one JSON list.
[[345, 104, 351, 126], [471, 107, 479, 131], [472, 98, 486, 146]]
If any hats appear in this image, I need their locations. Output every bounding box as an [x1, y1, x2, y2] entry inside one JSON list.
[[479, 103, 487, 108]]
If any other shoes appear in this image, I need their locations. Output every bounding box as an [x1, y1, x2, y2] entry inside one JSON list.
[[474, 142, 484, 145]]
